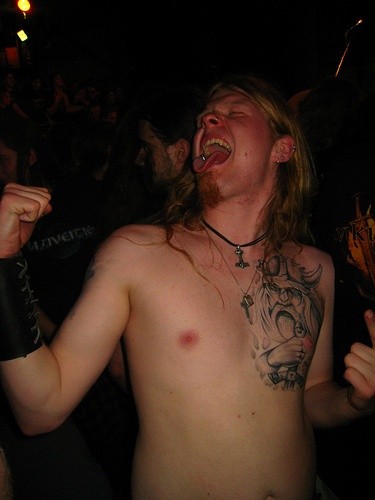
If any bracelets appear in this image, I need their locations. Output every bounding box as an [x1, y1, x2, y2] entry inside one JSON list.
[[346, 386, 374, 412]]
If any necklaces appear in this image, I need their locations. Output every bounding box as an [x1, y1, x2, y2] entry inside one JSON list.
[[200, 218, 270, 268], [201, 224, 264, 324]]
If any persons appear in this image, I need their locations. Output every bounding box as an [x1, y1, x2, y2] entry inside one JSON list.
[[0, 73, 375, 500], [0, 74, 375, 500]]
